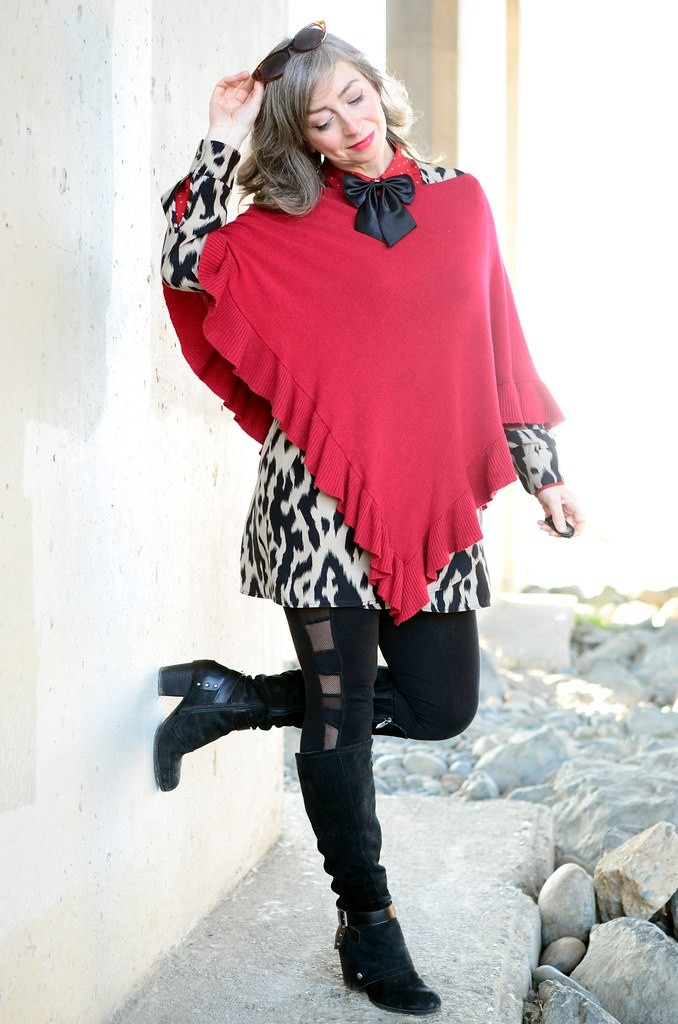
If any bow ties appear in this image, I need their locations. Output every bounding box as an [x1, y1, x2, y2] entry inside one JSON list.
[[342, 173, 418, 248]]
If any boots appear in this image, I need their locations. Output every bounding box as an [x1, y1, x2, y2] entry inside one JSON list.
[[153, 660, 406, 792], [295, 738, 441, 1015]]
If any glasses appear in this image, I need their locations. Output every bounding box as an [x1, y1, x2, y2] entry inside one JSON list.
[[252, 19, 327, 82]]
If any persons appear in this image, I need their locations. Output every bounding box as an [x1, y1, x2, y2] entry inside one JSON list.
[[152, 35, 584, 1016]]
